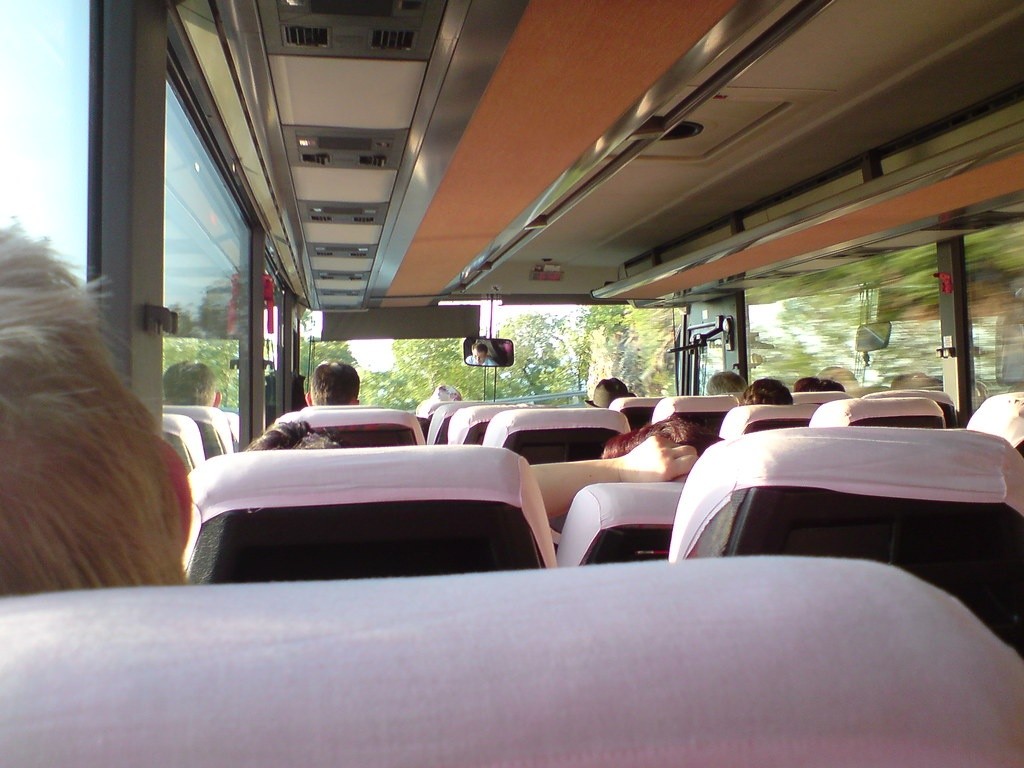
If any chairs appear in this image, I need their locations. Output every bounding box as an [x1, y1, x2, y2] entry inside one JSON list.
[[163, 404, 234, 459], [0, 391, 1024, 768], [224, 411, 239, 454], [162, 414, 205, 475]]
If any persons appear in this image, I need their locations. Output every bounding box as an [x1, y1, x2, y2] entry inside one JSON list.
[[466, 343, 498, 366], [0, 222, 191, 601], [706, 371, 845, 407], [831, 370, 943, 388], [245, 421, 339, 452], [305, 362, 359, 407], [584, 378, 636, 408], [163, 359, 221, 408], [529, 415, 725, 555]]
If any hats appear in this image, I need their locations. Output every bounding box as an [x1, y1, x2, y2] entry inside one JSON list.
[[416, 385, 461, 417], [585, 378, 637, 408]]
